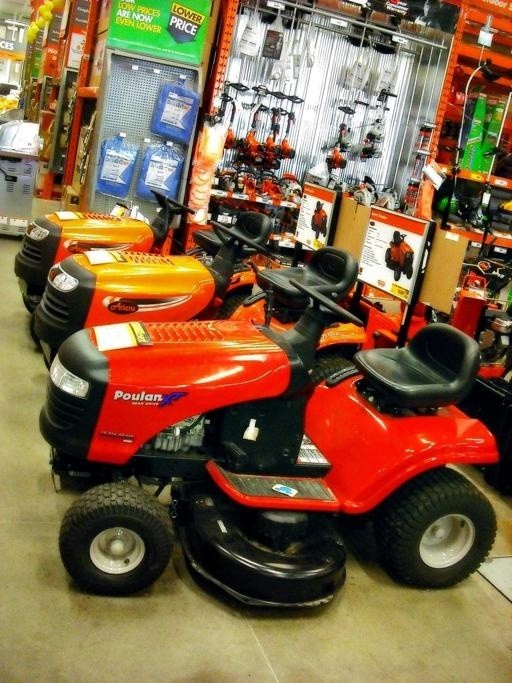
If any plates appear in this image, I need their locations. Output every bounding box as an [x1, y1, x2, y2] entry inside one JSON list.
[[435, 159, 511, 250]]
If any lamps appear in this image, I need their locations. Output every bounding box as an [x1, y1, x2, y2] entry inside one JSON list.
[[193, 212, 273, 255], [352, 322, 481, 411], [253, 245, 357, 319]]
